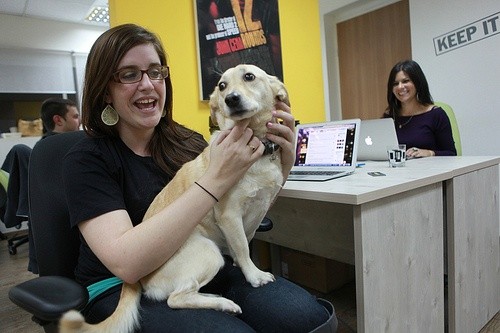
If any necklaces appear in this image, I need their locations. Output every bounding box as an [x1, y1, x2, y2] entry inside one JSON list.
[[397, 110, 413, 129]]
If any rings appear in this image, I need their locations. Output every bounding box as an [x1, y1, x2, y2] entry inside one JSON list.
[[248, 143, 256, 151], [413, 148, 418, 152]]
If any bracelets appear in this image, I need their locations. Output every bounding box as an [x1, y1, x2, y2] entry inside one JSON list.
[[194, 181, 220, 203]]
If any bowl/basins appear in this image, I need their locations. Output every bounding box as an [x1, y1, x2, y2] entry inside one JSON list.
[[1, 133, 22, 139]]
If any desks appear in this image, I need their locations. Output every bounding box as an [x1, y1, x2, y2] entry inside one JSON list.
[[255, 155, 500, 333]]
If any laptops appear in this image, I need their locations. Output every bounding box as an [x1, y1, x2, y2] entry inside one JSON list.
[[287, 118, 360, 182], [356, 119, 400, 161]]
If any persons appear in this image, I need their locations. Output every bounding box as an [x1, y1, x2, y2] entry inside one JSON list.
[[28, 97, 91, 276], [61, 24, 338, 333], [382, 60, 458, 157]]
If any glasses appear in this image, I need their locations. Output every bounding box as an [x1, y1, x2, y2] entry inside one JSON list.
[[111, 65, 169, 84]]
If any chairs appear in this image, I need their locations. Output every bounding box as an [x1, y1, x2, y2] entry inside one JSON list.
[[8, 131, 273, 333], [435, 102, 462, 156], [0, 144, 32, 255]]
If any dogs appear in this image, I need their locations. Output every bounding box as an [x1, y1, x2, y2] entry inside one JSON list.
[[58, 63, 298, 333]]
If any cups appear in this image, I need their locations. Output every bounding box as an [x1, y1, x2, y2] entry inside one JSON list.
[[10, 127, 17, 133], [387, 144, 407, 167]]
